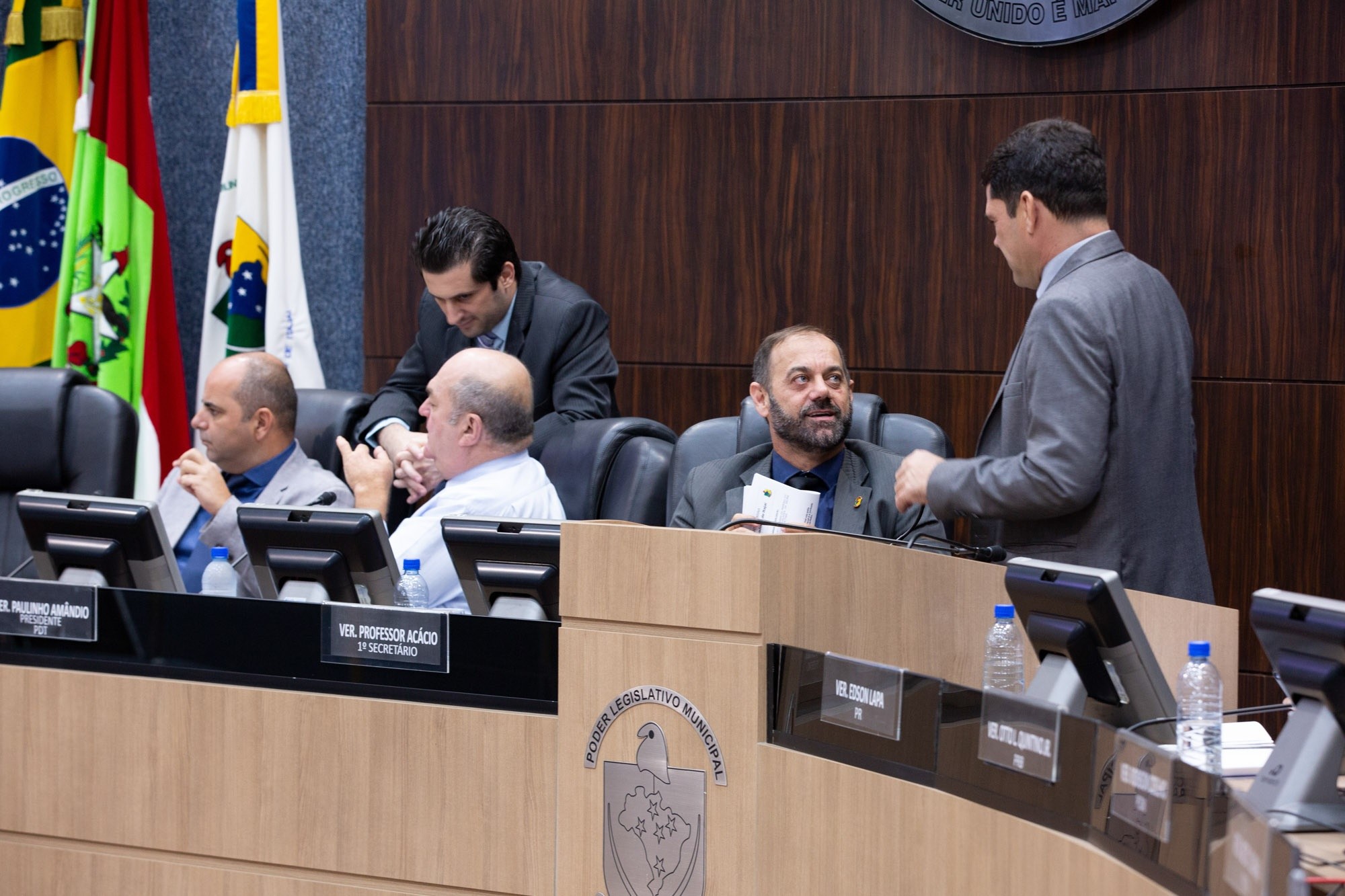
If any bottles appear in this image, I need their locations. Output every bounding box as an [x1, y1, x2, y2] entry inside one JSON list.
[[1175, 640, 1222, 775], [392, 558, 430, 610], [981, 604, 1024, 696], [201, 546, 237, 597]]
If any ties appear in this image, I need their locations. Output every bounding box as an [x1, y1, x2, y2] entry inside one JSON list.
[[475, 332, 497, 349], [180, 475, 244, 593], [787, 473, 824, 490]]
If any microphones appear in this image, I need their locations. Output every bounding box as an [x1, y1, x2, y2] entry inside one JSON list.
[[7, 489, 104, 577], [231, 491, 338, 568], [909, 532, 1007, 562]]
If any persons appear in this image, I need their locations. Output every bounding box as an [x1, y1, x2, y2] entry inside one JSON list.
[[152, 351, 355, 600], [669, 325, 951, 558], [354, 205, 619, 504], [894, 118, 1214, 603], [335, 347, 566, 617]]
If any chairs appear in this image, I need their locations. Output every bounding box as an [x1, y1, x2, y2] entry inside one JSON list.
[[534, 417, 680, 525], [290, 387, 378, 473], [0, 367, 139, 577], [665, 389, 955, 530]]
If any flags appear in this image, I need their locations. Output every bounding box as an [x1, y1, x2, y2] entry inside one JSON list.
[[1, 2, 329, 503]]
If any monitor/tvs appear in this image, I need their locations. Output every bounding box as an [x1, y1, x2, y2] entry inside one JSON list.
[[16, 489, 187, 593], [236, 502, 409, 607], [1234, 588, 1345, 834], [441, 515, 566, 622], [1003, 556, 1181, 745]]
